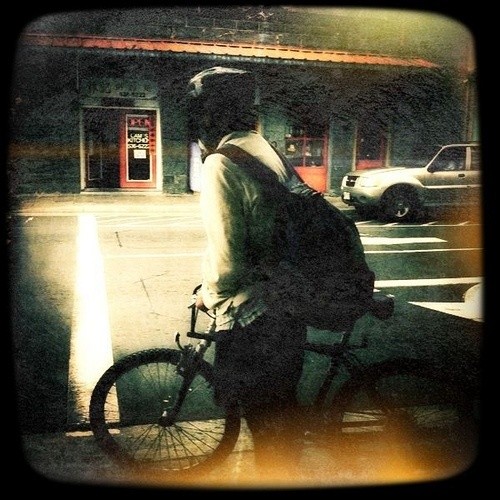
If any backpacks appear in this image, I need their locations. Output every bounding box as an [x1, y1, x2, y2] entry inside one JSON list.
[[201, 138, 373, 333]]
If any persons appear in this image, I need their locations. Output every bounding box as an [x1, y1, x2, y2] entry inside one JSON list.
[[187, 65, 308, 486]]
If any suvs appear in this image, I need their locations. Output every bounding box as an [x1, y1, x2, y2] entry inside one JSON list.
[[339, 141, 482, 225]]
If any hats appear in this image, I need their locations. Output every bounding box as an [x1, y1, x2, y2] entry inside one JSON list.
[[187, 66, 259, 106]]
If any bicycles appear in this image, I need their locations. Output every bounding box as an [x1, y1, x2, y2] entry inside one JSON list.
[[88, 283, 482, 482]]
